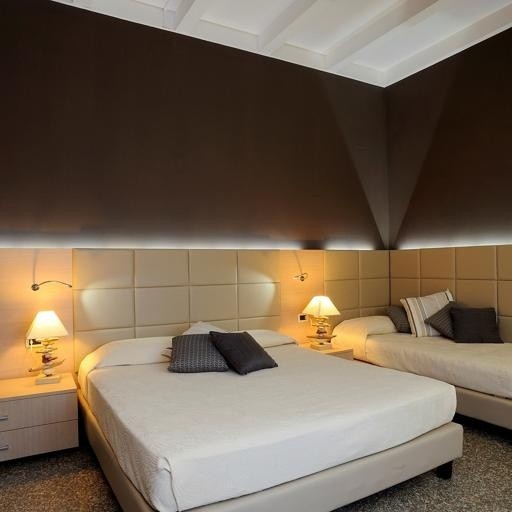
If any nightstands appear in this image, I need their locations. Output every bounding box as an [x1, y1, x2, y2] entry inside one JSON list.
[[298, 341, 353, 360], [1, 373, 80, 463]]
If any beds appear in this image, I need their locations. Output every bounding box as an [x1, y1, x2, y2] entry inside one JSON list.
[[73, 344, 463, 512], [331, 325, 512, 431]]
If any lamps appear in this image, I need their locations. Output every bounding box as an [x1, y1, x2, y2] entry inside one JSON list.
[[302, 296, 342, 349], [25, 310, 66, 385]]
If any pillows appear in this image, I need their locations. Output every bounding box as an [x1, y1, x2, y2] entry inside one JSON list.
[[161, 320, 278, 374], [382, 288, 504, 345]]
